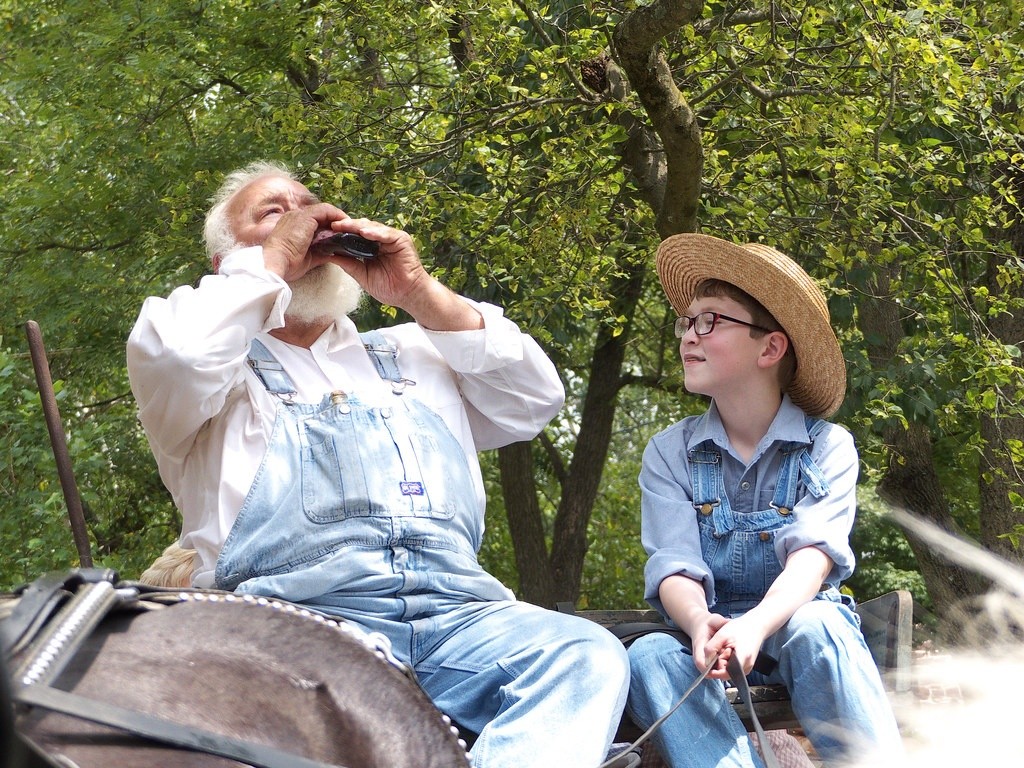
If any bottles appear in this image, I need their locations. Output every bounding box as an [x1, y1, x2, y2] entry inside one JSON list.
[[308, 234, 379, 261]]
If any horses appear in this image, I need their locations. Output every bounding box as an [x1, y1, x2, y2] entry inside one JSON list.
[[0, 587, 473, 768]]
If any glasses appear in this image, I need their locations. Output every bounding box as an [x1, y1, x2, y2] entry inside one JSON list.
[[674, 311, 774, 338]]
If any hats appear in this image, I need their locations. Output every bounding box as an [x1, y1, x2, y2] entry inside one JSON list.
[[655, 232, 847, 418]]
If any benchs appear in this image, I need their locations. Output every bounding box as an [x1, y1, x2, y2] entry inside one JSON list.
[[548, 589, 913, 767]]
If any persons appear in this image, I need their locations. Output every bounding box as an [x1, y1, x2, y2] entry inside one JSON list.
[[127, 160, 631, 768], [625, 233, 903, 768]]
